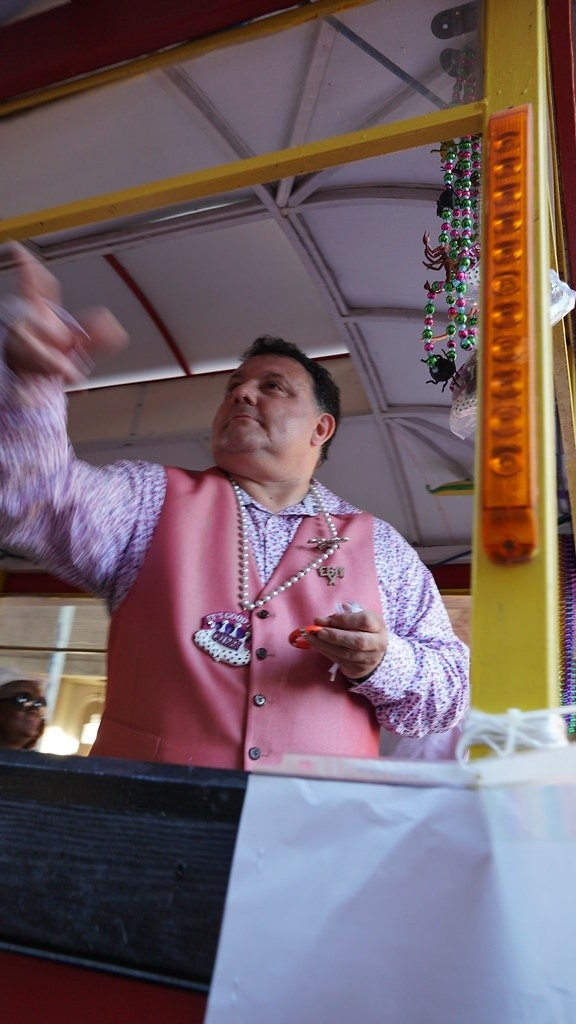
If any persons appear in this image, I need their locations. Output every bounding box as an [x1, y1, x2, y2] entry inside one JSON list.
[[0, 237, 476, 770], [0, 677, 48, 753]]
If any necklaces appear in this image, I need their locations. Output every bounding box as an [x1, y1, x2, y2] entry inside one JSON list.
[[193, 468, 341, 669]]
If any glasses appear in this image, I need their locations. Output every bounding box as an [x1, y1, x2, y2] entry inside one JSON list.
[[2, 691, 47, 710]]
